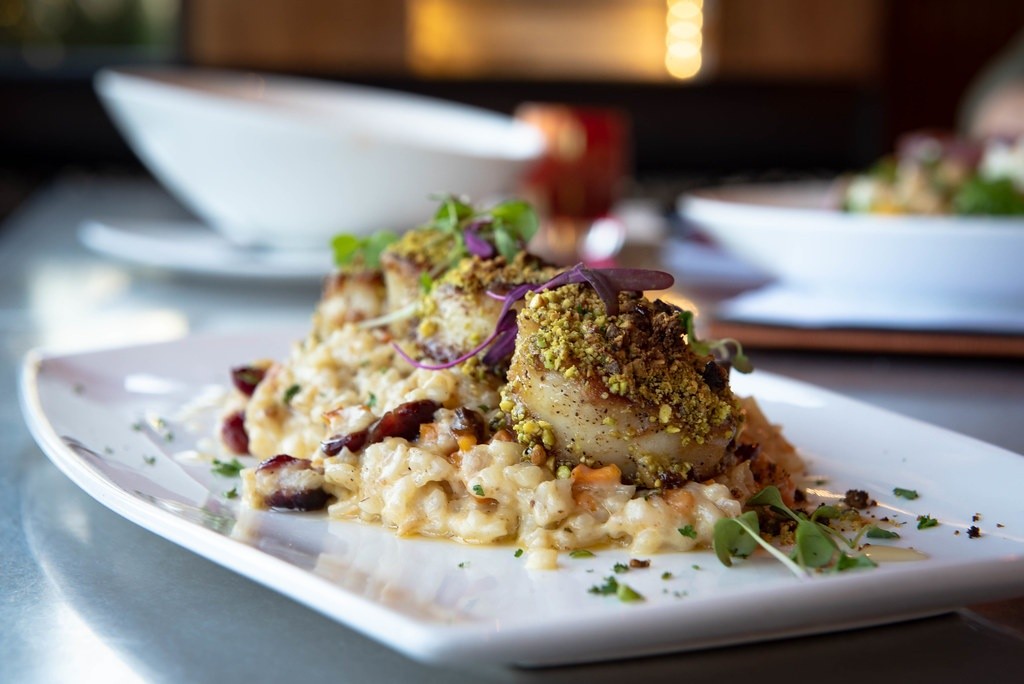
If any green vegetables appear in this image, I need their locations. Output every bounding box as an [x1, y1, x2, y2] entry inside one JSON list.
[[329, 197, 936, 603]]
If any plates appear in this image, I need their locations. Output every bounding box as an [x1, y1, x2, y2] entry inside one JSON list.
[[78, 217, 349, 292], [18, 322, 1024, 671]]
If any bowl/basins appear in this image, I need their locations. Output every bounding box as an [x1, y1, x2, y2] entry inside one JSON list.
[[680, 173, 1024, 303], [86, 65, 544, 259]]
